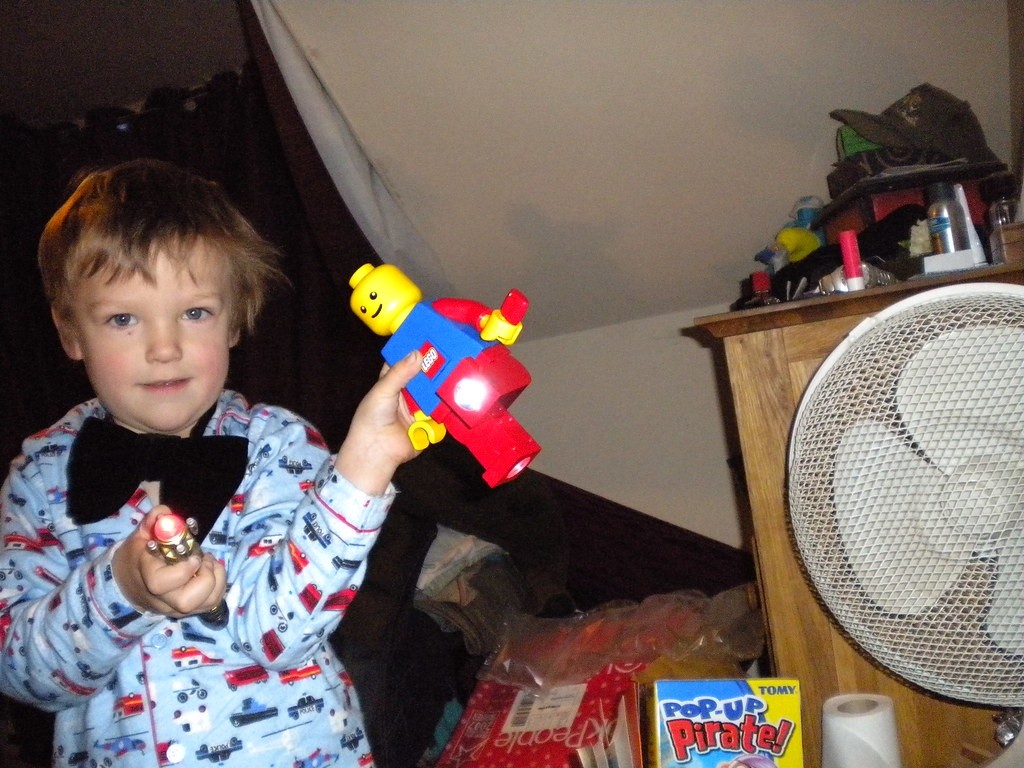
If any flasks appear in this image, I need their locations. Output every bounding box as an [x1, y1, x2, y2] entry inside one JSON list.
[[927, 181, 970, 257]]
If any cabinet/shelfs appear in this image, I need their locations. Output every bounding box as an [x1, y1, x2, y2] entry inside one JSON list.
[[692, 258, 1024, 768]]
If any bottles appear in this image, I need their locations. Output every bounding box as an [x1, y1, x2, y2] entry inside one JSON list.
[[984, 162, 1023, 230]]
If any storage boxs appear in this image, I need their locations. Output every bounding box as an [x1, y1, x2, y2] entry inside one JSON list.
[[836, 125, 883, 162], [645, 677, 803, 768]]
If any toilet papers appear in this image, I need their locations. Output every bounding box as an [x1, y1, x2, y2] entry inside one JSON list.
[[822, 694, 898, 768]]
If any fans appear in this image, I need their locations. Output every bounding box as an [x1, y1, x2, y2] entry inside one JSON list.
[[786, 282, 1024, 768]]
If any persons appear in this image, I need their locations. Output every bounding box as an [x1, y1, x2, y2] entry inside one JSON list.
[[0, 167, 425, 768]]
